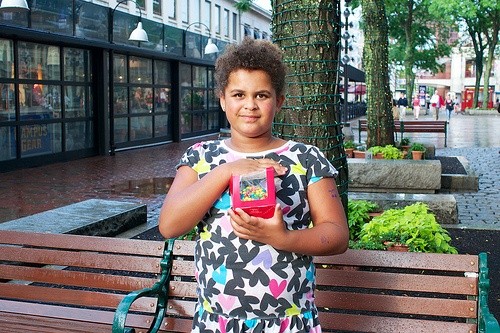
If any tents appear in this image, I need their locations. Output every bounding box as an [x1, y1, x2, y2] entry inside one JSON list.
[[338, 64, 365, 102], [348, 85, 366, 94]]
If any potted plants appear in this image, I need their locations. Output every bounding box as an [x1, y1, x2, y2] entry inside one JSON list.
[[354, 201, 458, 255], [343, 139, 426, 160]]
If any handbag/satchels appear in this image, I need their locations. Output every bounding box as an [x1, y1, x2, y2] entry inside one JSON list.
[[446, 104, 453, 111]]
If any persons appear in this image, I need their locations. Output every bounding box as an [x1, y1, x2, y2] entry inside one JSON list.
[[445, 94, 453, 120], [413, 96, 421, 120], [160, 37, 348, 333], [398, 92, 408, 121], [430, 90, 441, 121], [146, 89, 166, 113]]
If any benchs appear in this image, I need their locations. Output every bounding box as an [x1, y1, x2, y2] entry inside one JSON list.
[[359, 119, 449, 147], [1, 230, 499, 333], [348, 158, 442, 193]]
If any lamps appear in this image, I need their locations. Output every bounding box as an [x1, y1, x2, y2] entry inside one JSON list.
[[109, 0, 148, 42], [0, 0, 30, 12], [184, 22, 219, 54]]
